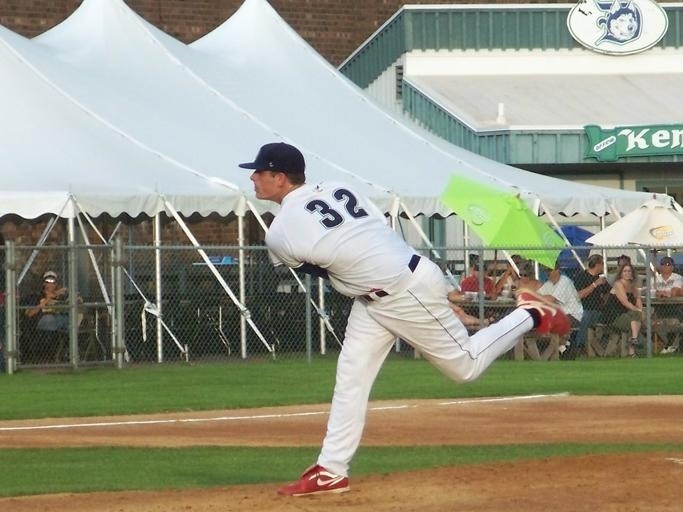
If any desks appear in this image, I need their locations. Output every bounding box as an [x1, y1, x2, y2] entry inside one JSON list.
[[43, 300, 139, 363], [451, 296, 683, 361]]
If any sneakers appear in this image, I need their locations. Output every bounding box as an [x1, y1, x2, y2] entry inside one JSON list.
[[276, 464, 349, 497], [516, 287, 569, 338], [562, 338, 679, 360]]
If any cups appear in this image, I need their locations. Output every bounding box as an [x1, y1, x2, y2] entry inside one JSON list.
[[502, 290, 509, 300], [599, 274, 605, 278], [650, 289, 657, 298], [466, 291, 479, 302]]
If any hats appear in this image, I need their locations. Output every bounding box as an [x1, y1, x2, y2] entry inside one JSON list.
[[470, 251, 559, 272], [43, 271, 57, 284], [660, 257, 674, 265], [238, 143, 305, 173]]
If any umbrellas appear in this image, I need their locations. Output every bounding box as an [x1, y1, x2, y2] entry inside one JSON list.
[[440, 171, 568, 301], [583, 193, 682, 355]]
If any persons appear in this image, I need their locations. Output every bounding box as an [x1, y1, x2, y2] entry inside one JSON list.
[[23, 270, 84, 360], [431, 253, 683, 360], [236, 141, 572, 499]]
